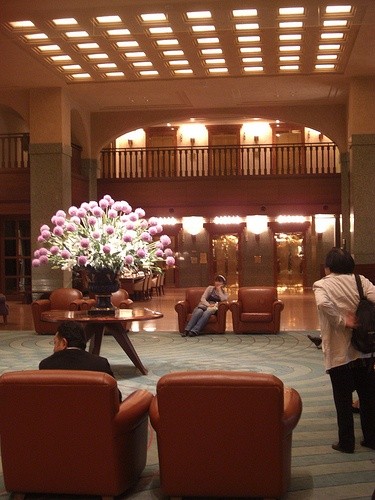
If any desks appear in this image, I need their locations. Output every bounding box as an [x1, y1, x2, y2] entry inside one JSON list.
[[119, 274, 158, 297], [41, 307, 164, 376]]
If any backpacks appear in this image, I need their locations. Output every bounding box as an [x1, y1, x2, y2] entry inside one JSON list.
[[351, 273, 375, 354]]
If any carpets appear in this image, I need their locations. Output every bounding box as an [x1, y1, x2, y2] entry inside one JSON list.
[[0, 330, 374, 500]]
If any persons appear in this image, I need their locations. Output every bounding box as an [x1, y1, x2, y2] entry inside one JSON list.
[[313, 247, 375, 453], [181, 273, 230, 338], [307, 332, 323, 351], [352, 397, 361, 414], [38, 319, 123, 400]]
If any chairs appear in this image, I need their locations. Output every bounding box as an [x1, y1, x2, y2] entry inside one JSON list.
[[134, 272, 166, 300]]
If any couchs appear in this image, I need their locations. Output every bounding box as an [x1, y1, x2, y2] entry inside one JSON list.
[[149, 372, 303, 500], [32, 288, 87, 334], [80, 289, 135, 335], [0, 370, 154, 500], [229, 286, 284, 334], [175, 287, 229, 334]]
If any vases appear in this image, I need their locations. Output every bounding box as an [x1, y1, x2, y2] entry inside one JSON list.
[[87, 269, 122, 316]]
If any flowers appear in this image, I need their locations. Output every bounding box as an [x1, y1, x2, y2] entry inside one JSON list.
[[32, 195, 175, 275]]
